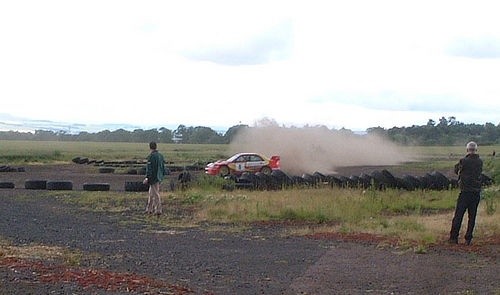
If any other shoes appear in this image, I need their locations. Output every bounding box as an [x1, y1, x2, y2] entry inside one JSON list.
[[153, 212, 162, 217], [463, 241, 470, 246], [448, 238, 458, 244], [142, 211, 152, 215]]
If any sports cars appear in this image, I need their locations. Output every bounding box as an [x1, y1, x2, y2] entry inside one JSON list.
[[203, 152, 282, 176]]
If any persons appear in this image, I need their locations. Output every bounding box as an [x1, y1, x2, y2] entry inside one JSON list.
[[178, 168, 192, 192], [448, 141, 483, 246], [143, 141, 165, 216]]
